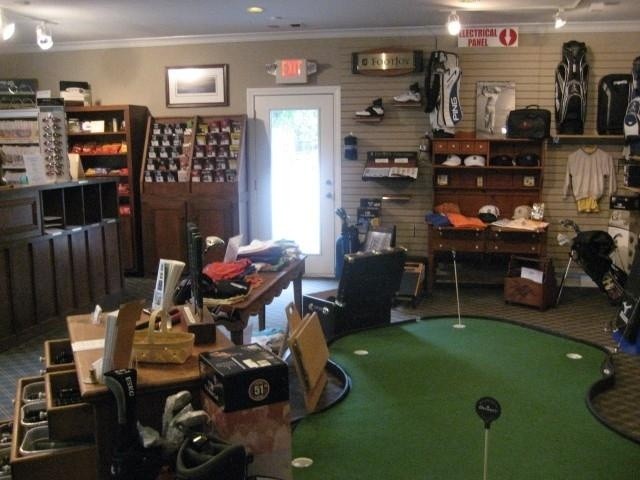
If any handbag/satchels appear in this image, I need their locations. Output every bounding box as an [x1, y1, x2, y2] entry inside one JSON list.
[[506, 104, 551, 139]]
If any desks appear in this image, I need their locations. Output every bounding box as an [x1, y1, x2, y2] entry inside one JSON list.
[[191, 252, 306, 346]]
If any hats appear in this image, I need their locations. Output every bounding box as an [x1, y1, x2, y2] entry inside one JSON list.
[[516, 151, 540, 166], [464, 155, 485, 167], [441, 154, 461, 166], [511, 205, 532, 219], [489, 155, 513, 166], [478, 204, 500, 218]]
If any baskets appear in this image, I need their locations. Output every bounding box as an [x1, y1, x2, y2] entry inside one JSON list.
[[131, 310, 195, 364]]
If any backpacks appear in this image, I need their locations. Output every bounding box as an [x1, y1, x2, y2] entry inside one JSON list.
[[622, 57, 640, 145], [554, 39, 590, 135], [596, 72, 633, 135]]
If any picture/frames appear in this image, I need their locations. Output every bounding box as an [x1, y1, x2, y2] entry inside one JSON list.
[[164, 64, 229, 108]]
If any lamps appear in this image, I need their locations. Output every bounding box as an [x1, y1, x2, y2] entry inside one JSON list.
[[446, 10, 462, 35], [1, 13, 16, 40], [552, 12, 565, 29], [37, 20, 54, 50]]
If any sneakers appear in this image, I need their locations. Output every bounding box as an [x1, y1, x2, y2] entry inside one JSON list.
[[355, 105, 385, 116], [393, 89, 422, 102]]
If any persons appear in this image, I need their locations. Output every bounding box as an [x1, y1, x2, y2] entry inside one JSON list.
[[482, 82, 502, 135]]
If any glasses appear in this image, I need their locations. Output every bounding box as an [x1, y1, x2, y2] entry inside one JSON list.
[[45, 147, 63, 152], [44, 140, 62, 146], [42, 115, 62, 122], [45, 162, 63, 167], [46, 170, 64, 176], [45, 155, 63, 161], [43, 132, 62, 137], [43, 124, 62, 130]]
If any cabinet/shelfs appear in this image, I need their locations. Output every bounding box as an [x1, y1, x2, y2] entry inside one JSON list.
[[140, 114, 249, 281], [65, 106, 147, 275], [0, 305, 241, 479], [428, 138, 546, 295], [0, 178, 123, 345]]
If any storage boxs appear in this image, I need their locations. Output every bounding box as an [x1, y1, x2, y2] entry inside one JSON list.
[[303, 246, 407, 337]]
